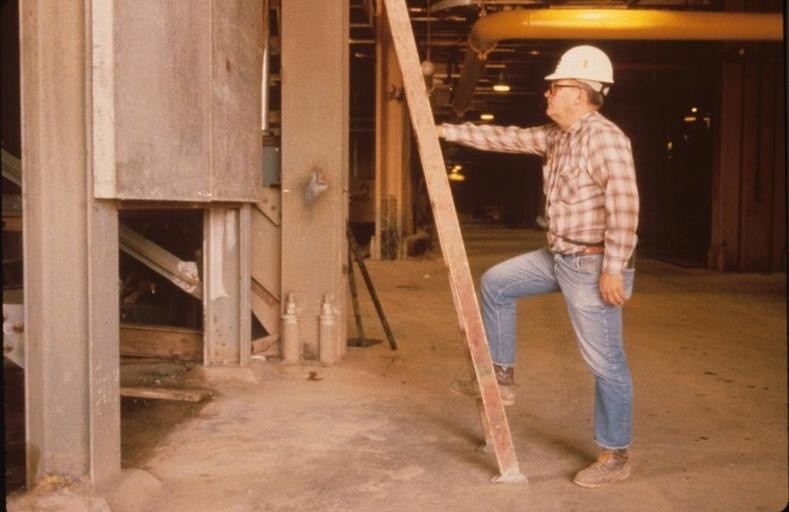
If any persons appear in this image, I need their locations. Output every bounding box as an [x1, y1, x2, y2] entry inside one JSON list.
[[434, 45, 641, 487]]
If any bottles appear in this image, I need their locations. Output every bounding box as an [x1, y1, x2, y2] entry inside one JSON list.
[[280, 292, 299, 364], [318, 294, 337, 366], [624, 248, 638, 270]]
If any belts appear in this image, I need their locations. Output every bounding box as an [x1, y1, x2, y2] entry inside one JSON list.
[[562, 245, 605, 257]]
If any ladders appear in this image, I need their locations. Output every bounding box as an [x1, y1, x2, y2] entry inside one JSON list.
[[382, 1, 524, 477]]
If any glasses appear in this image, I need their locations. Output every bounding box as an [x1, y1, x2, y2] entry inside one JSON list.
[[548, 84, 581, 95]]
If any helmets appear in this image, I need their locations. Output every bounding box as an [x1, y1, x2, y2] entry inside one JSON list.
[[544, 44, 615, 84]]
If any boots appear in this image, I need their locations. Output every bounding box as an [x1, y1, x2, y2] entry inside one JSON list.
[[573, 447, 631, 489], [449, 365, 516, 406]]
